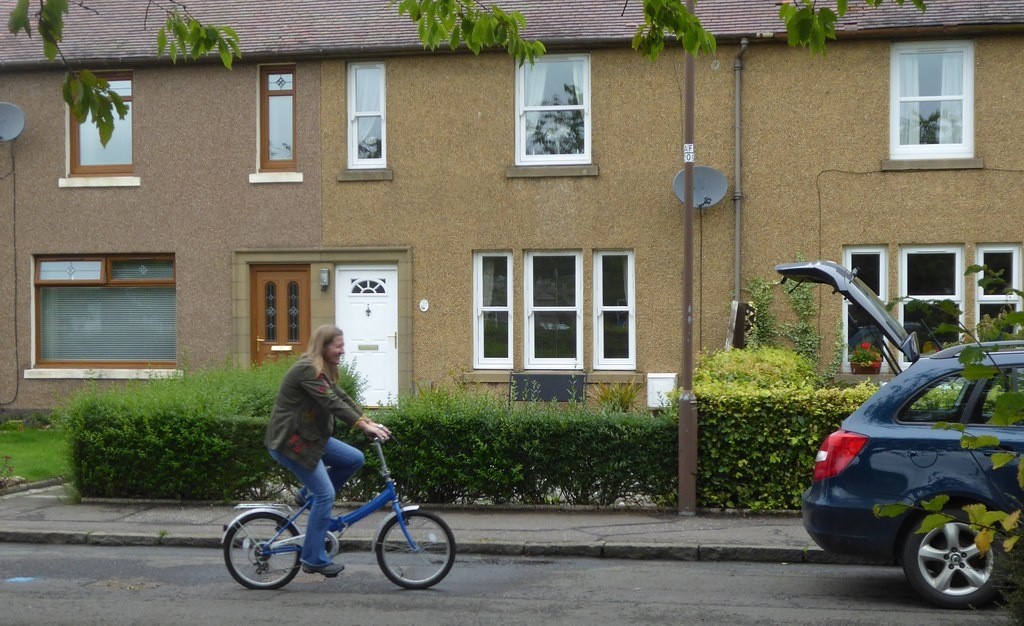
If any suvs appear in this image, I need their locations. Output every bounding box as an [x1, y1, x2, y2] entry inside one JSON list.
[[775, 261, 1024, 607]]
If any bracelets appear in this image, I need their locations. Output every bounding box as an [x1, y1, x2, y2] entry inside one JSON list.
[[366, 419, 371, 424]]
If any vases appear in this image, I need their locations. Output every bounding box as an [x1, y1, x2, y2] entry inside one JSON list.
[[850, 362, 882, 374]]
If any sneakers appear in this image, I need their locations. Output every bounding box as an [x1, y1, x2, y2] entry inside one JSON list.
[[301, 562, 345, 576], [294, 494, 310, 510]]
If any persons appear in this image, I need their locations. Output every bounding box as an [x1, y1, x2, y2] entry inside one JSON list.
[[265, 324, 392, 577]]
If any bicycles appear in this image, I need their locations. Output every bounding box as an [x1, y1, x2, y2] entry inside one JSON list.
[[219, 435, 456, 590]]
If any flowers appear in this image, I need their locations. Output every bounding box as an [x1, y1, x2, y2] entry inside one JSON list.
[[849, 342, 882, 368]]
